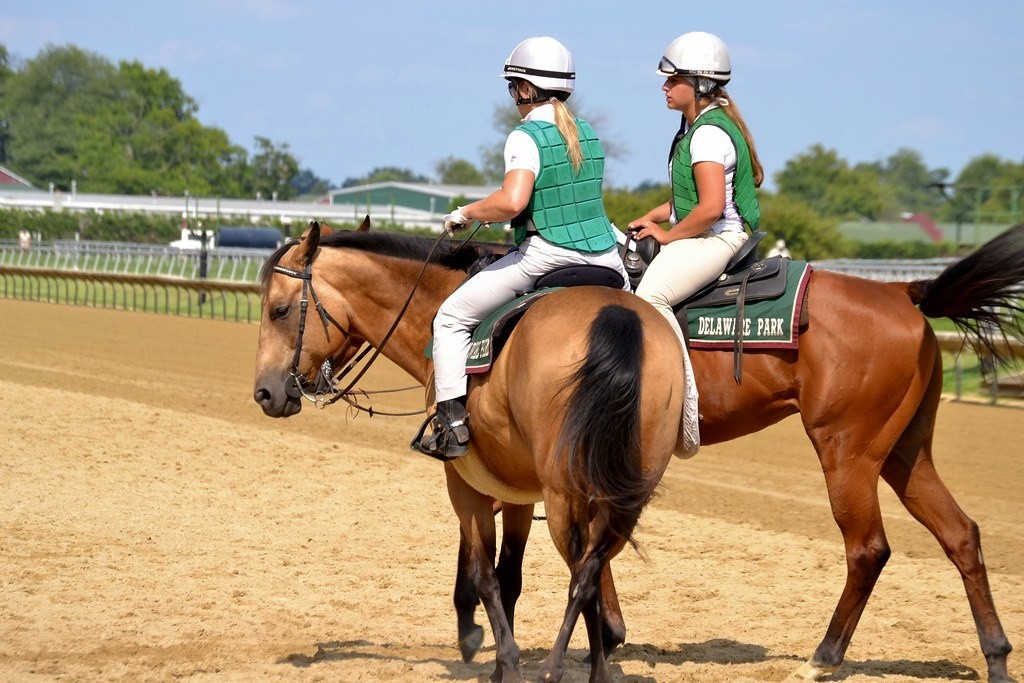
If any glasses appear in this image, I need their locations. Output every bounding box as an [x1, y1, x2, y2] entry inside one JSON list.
[[659, 56, 676, 74], [508, 83, 521, 98]]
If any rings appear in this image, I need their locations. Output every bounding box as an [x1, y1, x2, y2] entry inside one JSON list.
[[645, 222, 649, 227]]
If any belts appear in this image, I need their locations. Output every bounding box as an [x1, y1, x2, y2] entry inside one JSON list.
[[524, 230, 542, 237]]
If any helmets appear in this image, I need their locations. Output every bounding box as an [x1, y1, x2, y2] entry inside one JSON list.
[[500, 37, 575, 93], [656, 32, 731, 85]]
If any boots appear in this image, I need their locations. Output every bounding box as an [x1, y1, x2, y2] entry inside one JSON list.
[[422, 396, 470, 457]]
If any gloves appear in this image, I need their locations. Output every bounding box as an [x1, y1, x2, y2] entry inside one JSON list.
[[444, 205, 474, 238]]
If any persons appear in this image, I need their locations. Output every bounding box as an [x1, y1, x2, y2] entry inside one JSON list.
[[628, 31, 765, 398], [419, 35, 636, 458]]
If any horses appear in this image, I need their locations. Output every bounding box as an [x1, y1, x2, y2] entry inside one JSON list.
[[253, 215, 684, 683], [307, 215, 1024, 683]]
